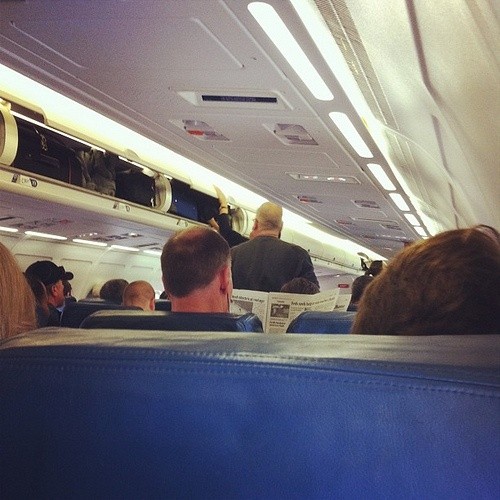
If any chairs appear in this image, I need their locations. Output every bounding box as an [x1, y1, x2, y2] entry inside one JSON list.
[[1, 299, 500, 500]]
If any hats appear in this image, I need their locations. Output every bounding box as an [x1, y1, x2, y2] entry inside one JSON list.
[[26, 260, 73, 285]]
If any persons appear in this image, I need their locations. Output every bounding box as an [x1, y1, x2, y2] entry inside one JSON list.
[[0, 242, 156, 344], [160, 186, 320, 313], [347, 224, 500, 335]]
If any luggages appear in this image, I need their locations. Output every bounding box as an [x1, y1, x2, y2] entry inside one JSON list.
[[12, 119, 233, 234]]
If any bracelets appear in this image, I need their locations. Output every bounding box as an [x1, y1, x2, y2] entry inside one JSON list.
[[219, 206, 228, 210]]
